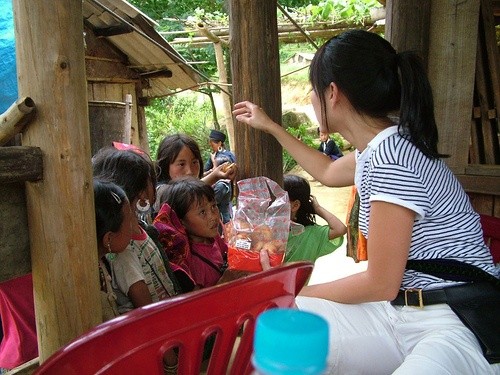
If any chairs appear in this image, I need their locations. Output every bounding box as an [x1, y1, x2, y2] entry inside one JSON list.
[[32, 259, 313, 375]]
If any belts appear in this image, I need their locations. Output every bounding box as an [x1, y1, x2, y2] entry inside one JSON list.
[[390, 286, 446, 309]]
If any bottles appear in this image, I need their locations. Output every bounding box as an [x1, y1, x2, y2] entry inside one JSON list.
[[249, 308, 330, 375]]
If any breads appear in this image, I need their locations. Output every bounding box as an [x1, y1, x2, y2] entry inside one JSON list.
[[228, 221, 286, 255], [224, 163, 237, 179]]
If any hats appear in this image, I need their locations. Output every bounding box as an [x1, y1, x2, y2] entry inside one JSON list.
[[209, 130, 226, 142]]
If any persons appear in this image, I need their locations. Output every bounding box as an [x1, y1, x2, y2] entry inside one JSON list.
[[232, 32, 500, 375], [0, 128, 240, 373], [283, 174, 346, 261], [307, 127, 344, 186]]
